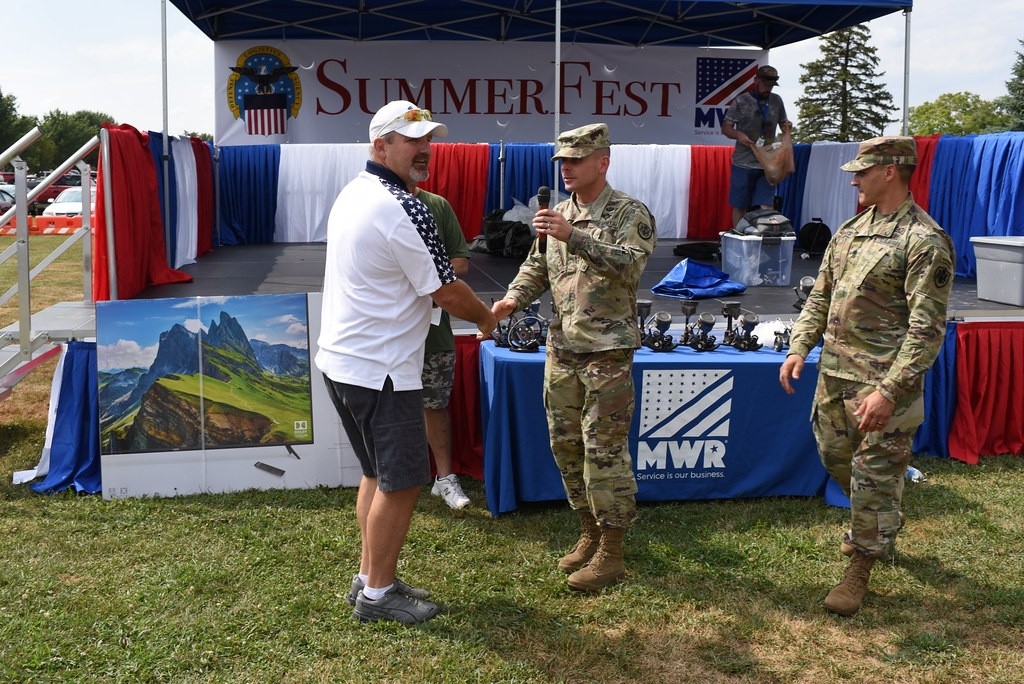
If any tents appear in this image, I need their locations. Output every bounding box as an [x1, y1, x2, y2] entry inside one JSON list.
[[161, 0, 913, 268]]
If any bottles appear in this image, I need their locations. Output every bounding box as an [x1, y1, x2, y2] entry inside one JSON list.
[[905, 465, 923, 483]]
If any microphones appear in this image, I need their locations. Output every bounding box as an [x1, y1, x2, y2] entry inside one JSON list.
[[537, 186, 551, 254]]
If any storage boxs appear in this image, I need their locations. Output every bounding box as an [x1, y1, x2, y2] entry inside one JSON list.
[[969, 236, 1024, 306], [719, 232, 796, 287]]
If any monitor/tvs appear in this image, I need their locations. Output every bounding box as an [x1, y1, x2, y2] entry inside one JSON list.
[[95, 292, 314, 461]]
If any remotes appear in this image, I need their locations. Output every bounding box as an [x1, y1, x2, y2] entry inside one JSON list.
[[254, 462, 285, 477]]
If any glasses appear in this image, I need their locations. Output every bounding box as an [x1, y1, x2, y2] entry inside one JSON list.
[[764, 76, 780, 82], [376, 108, 433, 137]]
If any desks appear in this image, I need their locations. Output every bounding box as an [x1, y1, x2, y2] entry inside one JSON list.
[[480, 338, 820, 499]]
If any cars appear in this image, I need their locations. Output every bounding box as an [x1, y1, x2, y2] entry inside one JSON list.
[[43, 186, 95, 217], [0, 172, 71, 217]]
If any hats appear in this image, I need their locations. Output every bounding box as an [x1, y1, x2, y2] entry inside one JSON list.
[[841, 136, 917, 172], [756, 65, 779, 87], [551, 123, 611, 162], [370, 100, 448, 143]]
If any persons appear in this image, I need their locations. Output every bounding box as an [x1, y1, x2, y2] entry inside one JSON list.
[[491, 122, 657, 590], [778, 136, 955, 618], [722, 65, 792, 229], [314, 100, 497, 625]]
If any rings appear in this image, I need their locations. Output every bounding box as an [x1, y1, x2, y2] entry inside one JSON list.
[[876, 422, 883, 426], [548, 222, 550, 228]]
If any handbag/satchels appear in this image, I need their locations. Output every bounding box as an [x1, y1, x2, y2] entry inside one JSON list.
[[750, 126, 795, 186]]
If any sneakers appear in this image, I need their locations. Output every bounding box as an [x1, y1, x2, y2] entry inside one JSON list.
[[431, 473, 470, 510], [352, 582, 439, 624], [346, 573, 432, 607]]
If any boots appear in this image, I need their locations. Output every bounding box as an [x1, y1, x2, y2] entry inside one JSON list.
[[824, 548, 876, 616], [558, 515, 603, 572], [567, 525, 627, 591], [841, 511, 906, 556]]
